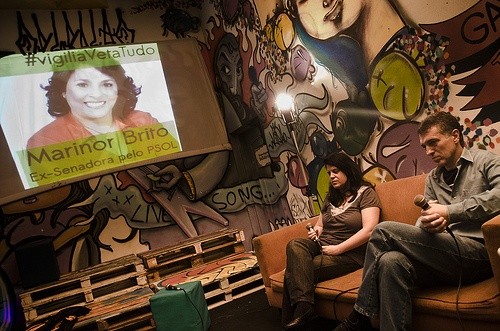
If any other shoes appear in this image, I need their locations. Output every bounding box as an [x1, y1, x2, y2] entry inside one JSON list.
[[283, 304, 318, 331], [334, 317, 372, 331]]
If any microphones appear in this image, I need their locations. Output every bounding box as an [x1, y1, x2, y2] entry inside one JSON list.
[[305, 223, 321, 247], [414, 194, 451, 234], [166, 283, 182, 290]]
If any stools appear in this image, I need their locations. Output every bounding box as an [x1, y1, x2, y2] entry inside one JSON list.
[[149, 281, 211, 331]]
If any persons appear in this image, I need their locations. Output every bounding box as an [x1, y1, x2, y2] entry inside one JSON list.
[[281, 152, 382, 331], [334, 111, 500, 331], [26, 49, 180, 187]]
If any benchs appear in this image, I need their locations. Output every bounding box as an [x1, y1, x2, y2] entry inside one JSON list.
[[19, 229, 265, 331]]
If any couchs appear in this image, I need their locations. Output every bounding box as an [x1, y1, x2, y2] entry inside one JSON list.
[[252, 174, 500, 331]]
